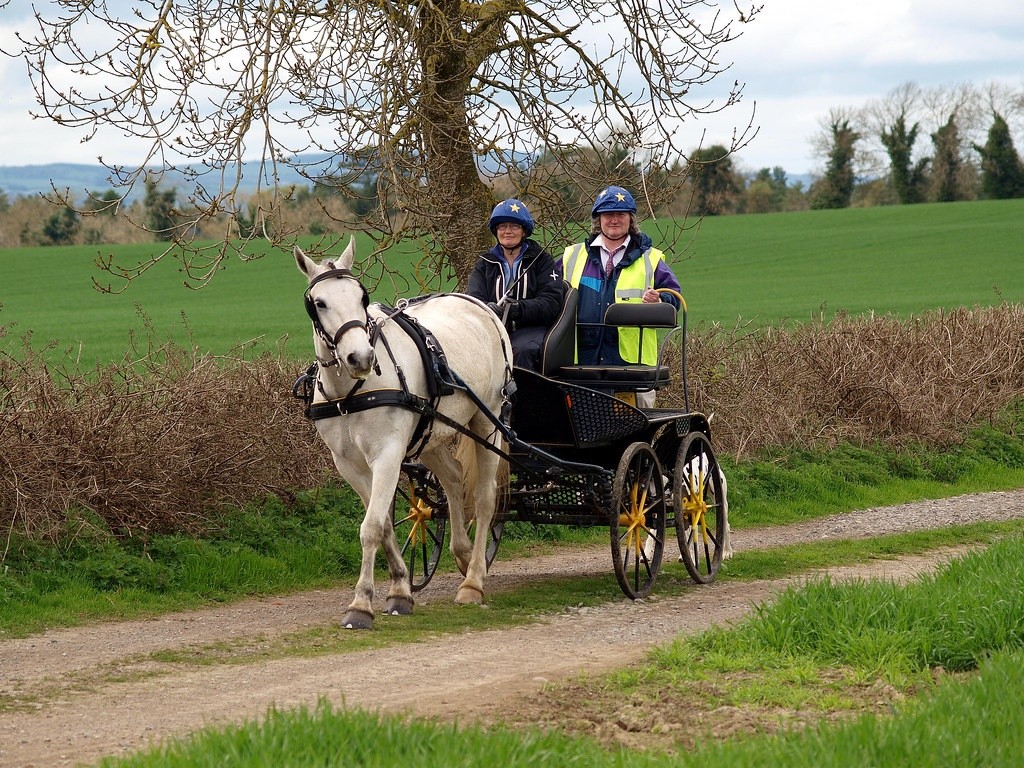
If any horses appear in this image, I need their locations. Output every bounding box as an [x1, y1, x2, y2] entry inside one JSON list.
[[293, 233, 513, 630]]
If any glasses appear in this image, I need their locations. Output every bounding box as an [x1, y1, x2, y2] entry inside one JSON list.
[[498, 223, 523, 231]]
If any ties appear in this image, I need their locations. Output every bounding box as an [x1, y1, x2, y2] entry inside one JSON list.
[[601, 245, 626, 279]]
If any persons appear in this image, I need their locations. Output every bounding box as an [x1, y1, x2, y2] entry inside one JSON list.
[[467, 198, 564, 375], [555, 186, 680, 409]]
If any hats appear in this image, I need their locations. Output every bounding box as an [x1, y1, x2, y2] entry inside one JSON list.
[[488, 199, 533, 237], [592, 186, 636, 220]]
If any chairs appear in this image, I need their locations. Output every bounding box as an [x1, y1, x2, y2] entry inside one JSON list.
[[537, 277, 580, 380], [561, 299, 682, 394]]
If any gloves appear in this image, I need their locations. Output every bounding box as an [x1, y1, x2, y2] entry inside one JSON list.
[[487, 298, 521, 319]]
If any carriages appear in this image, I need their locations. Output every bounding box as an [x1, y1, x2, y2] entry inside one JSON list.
[[291, 234, 727, 632]]
